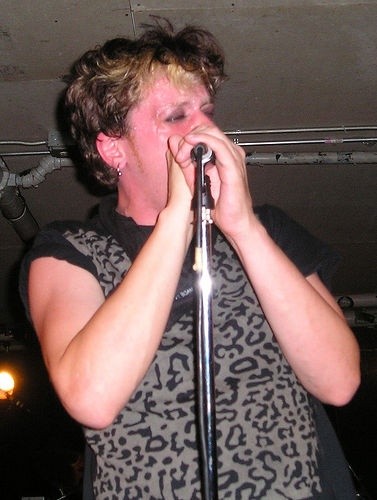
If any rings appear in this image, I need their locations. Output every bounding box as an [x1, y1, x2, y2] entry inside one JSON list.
[[233, 138, 239, 145]]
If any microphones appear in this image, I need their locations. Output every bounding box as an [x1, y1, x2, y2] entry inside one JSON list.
[[194, 143, 214, 163]]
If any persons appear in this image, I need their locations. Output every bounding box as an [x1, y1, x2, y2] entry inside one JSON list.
[[19, 14, 363, 500]]
